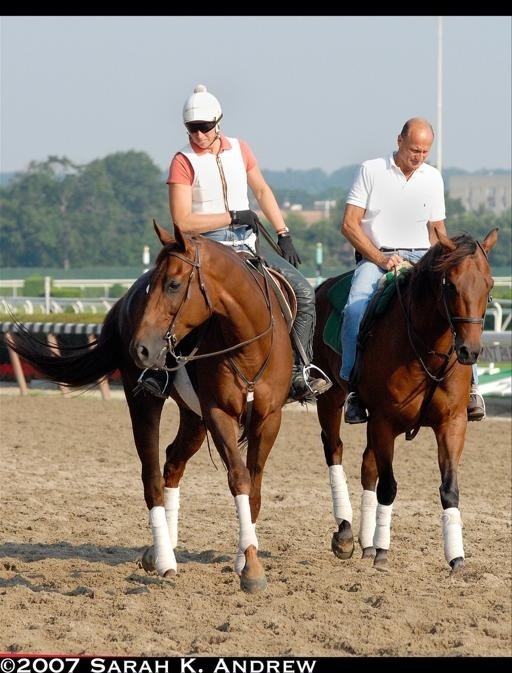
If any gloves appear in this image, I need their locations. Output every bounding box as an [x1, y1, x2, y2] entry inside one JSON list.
[[232, 208, 261, 229], [279, 231, 302, 265]]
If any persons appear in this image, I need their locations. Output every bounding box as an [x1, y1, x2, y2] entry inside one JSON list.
[[132, 81, 335, 405], [342, 115, 488, 426]]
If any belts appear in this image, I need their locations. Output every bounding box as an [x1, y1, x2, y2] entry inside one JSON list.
[[358, 244, 430, 255]]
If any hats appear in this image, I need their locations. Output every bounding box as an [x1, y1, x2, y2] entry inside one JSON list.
[[179, 85, 224, 124]]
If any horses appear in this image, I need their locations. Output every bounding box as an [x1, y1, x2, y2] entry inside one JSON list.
[[4, 218, 295, 597], [314, 227, 499, 577]]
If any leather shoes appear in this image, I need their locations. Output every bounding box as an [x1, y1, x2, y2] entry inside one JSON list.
[[138, 362, 171, 402], [285, 368, 331, 406], [467, 386, 488, 417], [343, 393, 365, 423]]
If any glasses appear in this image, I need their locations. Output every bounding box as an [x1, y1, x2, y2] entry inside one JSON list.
[[186, 115, 224, 131]]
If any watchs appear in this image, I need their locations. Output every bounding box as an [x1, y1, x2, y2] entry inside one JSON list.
[[276, 226, 290, 234]]
